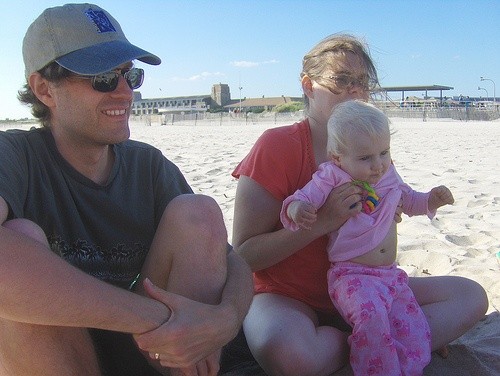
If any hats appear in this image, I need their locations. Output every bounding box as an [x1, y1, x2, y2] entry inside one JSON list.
[[22, 2, 161, 83]]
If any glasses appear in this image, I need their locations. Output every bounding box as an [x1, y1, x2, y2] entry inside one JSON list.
[[308, 73, 378, 92], [66, 66, 144, 91]]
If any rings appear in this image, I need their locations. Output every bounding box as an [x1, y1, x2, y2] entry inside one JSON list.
[[154, 353, 160, 359]]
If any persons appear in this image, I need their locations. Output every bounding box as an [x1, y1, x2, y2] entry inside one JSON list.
[[280, 100, 454, 376], [0, 3, 254, 376], [231, 35, 488, 376]]
